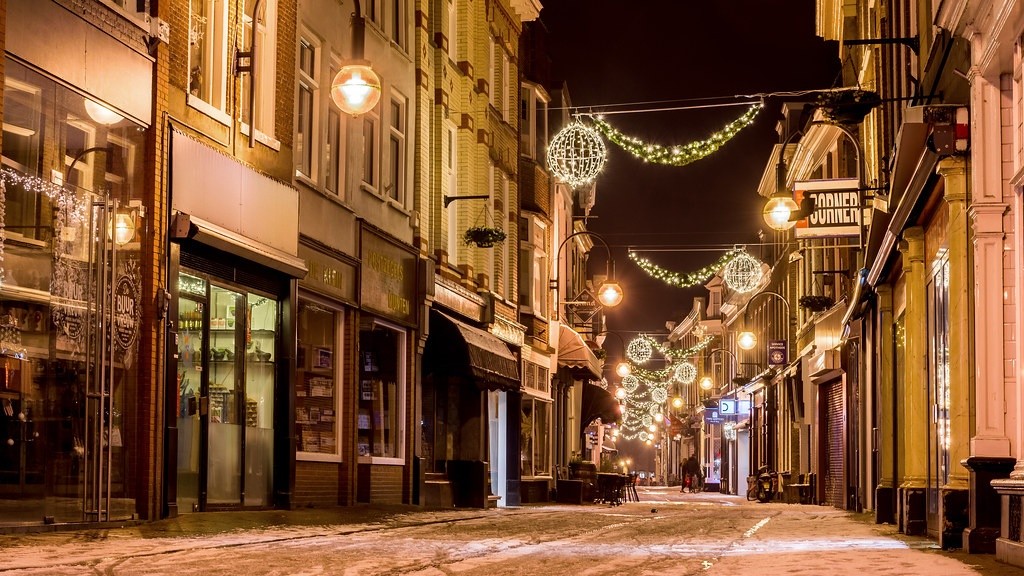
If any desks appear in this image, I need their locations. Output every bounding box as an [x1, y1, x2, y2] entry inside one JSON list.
[[177, 416, 271, 510], [594, 472, 629, 507]]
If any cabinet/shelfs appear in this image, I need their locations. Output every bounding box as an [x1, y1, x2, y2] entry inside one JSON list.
[[176, 329, 274, 365], [294, 396, 334, 426]]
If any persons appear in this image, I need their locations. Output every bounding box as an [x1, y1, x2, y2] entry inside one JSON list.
[[680, 454, 700, 493]]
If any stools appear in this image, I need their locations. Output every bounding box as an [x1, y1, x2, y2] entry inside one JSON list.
[[788, 483, 809, 503]]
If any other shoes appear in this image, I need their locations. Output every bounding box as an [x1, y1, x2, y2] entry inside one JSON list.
[[680, 490, 686, 493], [689, 490, 694, 493]]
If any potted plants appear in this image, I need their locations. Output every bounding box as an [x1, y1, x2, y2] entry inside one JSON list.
[[813, 51, 884, 125], [731, 370, 752, 386], [798, 276, 836, 312], [460, 208, 507, 248]]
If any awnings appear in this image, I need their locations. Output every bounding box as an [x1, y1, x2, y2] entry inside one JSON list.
[[431, 307, 521, 392], [583, 384, 621, 435], [558, 324, 602, 381]]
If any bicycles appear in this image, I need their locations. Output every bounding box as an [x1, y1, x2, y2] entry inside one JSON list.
[[746, 462, 776, 503], [693, 466, 705, 493]]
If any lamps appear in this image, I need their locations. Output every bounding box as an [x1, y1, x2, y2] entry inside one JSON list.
[[82, 97, 125, 126], [762, 119, 866, 277], [736, 291, 793, 365], [62, 144, 135, 249], [647, 350, 740, 447], [234, 0, 381, 150]]
